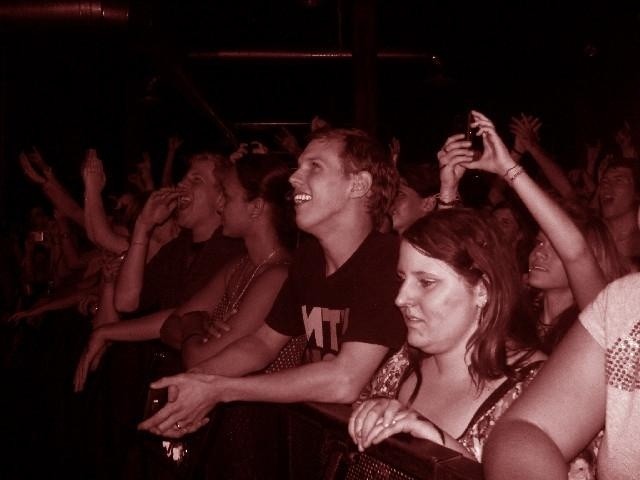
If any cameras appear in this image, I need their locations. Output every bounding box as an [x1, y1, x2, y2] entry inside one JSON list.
[[466, 113, 474, 141]]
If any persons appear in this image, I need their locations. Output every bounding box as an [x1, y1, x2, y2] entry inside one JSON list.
[[1, 93, 640, 480]]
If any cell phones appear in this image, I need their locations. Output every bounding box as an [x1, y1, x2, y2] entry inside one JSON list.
[[143, 386, 168, 417]]
